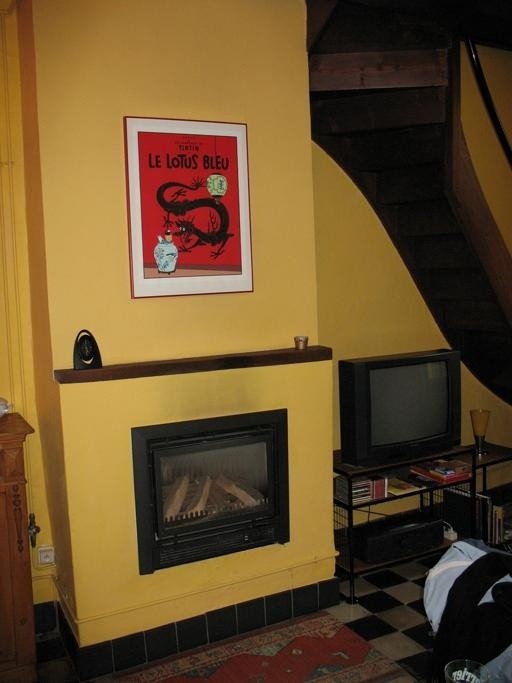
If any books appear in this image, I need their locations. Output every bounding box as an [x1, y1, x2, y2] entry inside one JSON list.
[[333, 455, 506, 545]]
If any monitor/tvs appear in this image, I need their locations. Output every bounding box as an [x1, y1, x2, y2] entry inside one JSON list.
[[338, 349, 462, 470]]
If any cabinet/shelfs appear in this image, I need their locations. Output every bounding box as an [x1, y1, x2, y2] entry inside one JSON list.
[[0, 411, 38, 682]]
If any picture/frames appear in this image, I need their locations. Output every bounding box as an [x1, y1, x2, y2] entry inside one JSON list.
[[124, 116, 254, 299]]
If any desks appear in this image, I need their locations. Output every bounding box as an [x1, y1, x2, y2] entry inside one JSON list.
[[333, 437, 512, 604]]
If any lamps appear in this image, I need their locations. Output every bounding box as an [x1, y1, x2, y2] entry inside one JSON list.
[[470, 409, 490, 456]]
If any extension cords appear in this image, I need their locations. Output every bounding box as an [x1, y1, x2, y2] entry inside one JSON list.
[[444, 527, 458, 541]]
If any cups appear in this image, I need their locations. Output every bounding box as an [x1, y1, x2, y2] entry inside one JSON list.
[[444, 659, 488, 682], [469, 408, 491, 452]]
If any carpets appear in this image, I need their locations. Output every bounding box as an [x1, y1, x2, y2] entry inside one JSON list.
[[115, 610, 418, 682]]
